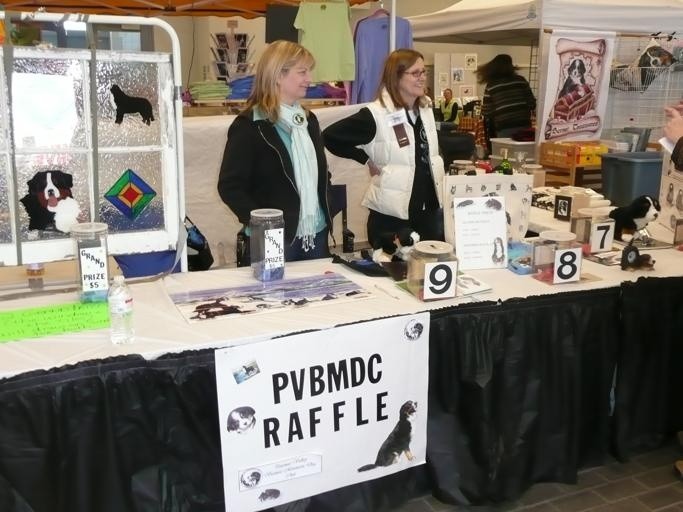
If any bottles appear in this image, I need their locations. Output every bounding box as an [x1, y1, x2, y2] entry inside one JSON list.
[[70, 222, 112, 303], [108, 276, 135, 349], [450, 148, 512, 176], [249, 208, 283, 281], [575, 207, 615, 255], [407, 241, 459, 302], [532, 230, 584, 284]]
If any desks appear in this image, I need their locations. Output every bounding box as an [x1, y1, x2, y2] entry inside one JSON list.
[[1, 250, 683, 512]]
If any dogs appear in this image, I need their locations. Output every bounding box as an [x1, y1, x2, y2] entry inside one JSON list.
[[639, 46, 675, 90], [18, 170, 74, 241], [357, 400, 419, 472], [557, 59, 587, 97], [227, 406, 256, 434], [370, 228, 422, 268]]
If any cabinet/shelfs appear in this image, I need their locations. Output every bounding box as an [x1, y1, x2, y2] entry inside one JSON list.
[[542, 138, 604, 195]]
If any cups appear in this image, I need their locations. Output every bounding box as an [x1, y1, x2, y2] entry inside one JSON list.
[[26, 263, 45, 280]]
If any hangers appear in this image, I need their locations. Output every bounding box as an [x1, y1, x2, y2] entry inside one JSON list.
[[366, 0, 393, 19]]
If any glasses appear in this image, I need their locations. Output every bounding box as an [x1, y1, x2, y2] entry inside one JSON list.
[[400, 69, 427, 78]]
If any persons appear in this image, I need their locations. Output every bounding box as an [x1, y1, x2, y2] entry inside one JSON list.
[[661, 100, 683, 171], [216, 39, 339, 262], [433, 88, 460, 127], [319, 47, 447, 250], [471, 54, 537, 138]]
